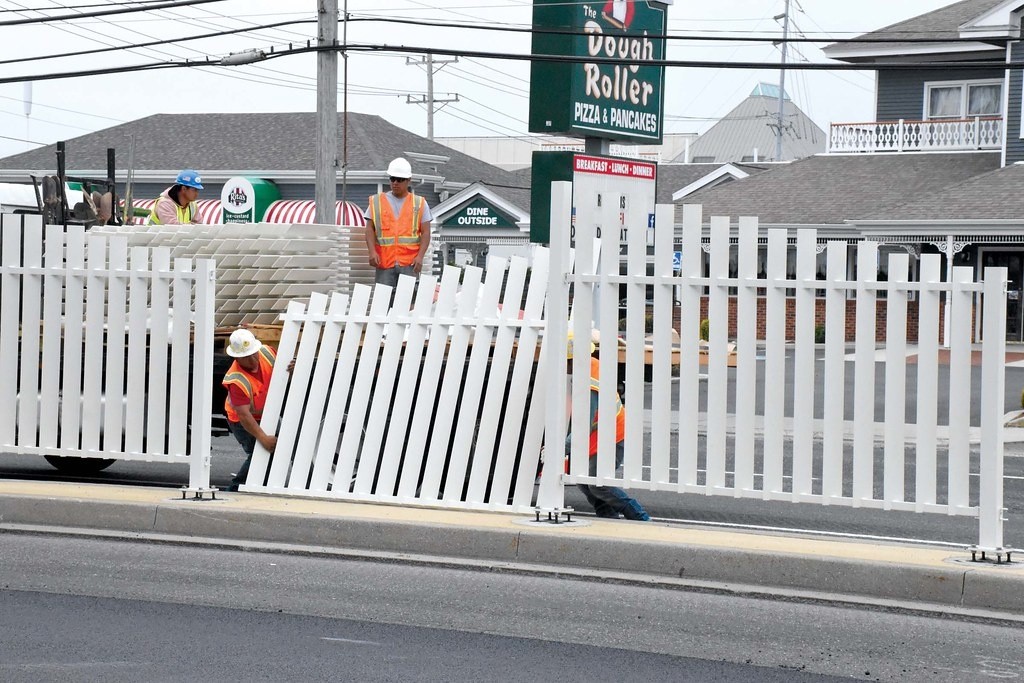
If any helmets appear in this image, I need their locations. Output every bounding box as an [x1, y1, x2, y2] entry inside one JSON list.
[[227, 329, 262, 358], [176, 170, 204, 189], [386, 157, 412, 178], [566, 320, 595, 359]]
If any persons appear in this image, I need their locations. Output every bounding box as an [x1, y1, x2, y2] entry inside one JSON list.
[[222, 329, 296, 491], [541, 333, 649, 520], [364, 159, 434, 309], [146, 171, 204, 225]]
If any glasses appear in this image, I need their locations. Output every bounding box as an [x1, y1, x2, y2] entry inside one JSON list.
[[390, 176, 410, 183]]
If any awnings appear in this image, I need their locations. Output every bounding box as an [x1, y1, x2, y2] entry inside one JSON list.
[[263, 199, 366, 227], [114, 198, 222, 225]]
